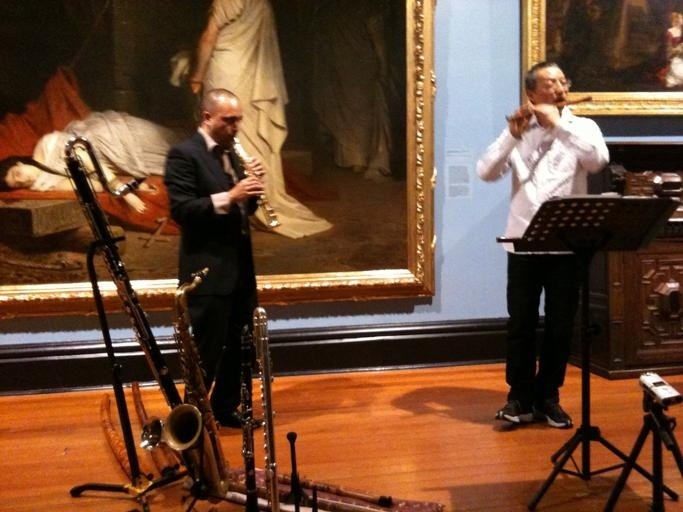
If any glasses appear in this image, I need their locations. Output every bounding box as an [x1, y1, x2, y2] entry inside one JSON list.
[[542, 80, 572, 90]]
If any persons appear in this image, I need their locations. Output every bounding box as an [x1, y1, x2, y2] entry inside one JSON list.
[[0, 110, 179, 214], [657, 12, 682, 88], [189, 1, 334, 239], [163, 89, 267, 431], [475, 63, 611, 428]]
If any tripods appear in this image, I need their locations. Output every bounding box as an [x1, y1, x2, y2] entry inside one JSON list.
[[526, 249, 679, 512], [604, 401, 683, 512], [70, 235, 188, 512]]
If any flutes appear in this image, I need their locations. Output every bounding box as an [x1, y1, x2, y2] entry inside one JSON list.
[[505, 96, 591, 121]]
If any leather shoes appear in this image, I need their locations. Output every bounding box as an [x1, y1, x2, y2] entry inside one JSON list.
[[213, 410, 262, 431]]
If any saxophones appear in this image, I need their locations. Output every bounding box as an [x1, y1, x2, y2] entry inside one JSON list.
[[163, 267, 229, 505]]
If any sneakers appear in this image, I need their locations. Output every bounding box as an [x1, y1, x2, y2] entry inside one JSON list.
[[495, 402, 535, 423], [532, 404, 572, 428]]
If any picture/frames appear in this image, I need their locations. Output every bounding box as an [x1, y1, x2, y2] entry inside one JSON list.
[[0, 0, 436, 321], [519, 0, 683, 116]]
